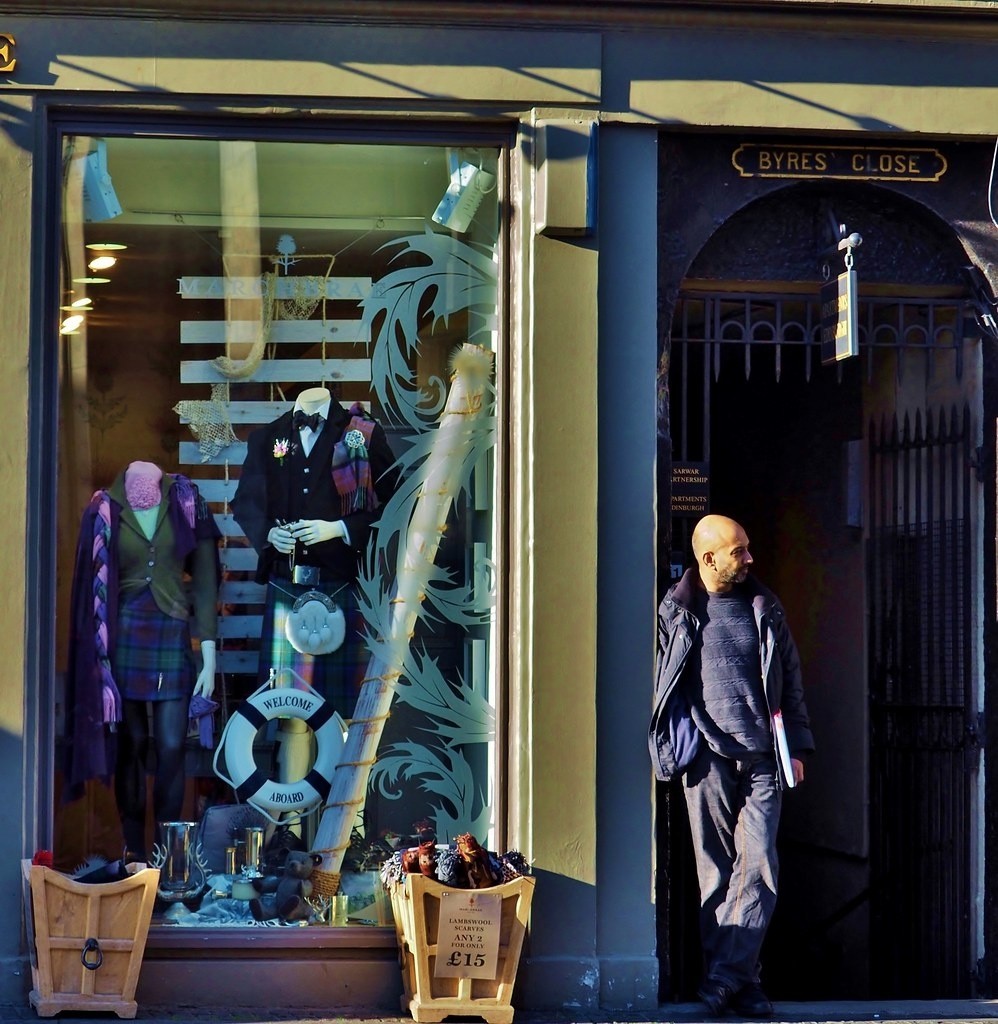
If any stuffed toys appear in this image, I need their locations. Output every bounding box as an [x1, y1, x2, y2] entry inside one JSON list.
[[249, 849, 323, 922]]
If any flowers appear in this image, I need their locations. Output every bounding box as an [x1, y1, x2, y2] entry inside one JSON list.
[[273, 438, 289, 466]]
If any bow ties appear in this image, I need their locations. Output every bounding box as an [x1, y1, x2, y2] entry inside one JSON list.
[[294, 410, 319, 433]]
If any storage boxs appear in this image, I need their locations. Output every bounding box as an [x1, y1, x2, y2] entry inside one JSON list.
[[388, 872, 536, 1023], [21, 858, 160, 1017]]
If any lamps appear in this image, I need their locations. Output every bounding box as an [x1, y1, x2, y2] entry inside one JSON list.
[[61, 137, 123, 222], [432, 145, 496, 233]]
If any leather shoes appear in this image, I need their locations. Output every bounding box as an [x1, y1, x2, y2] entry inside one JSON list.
[[696, 978, 774, 1018]]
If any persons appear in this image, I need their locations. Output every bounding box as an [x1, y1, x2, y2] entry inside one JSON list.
[[656, 515, 819, 1012], [230, 385, 406, 854], [68, 460, 218, 860]]
